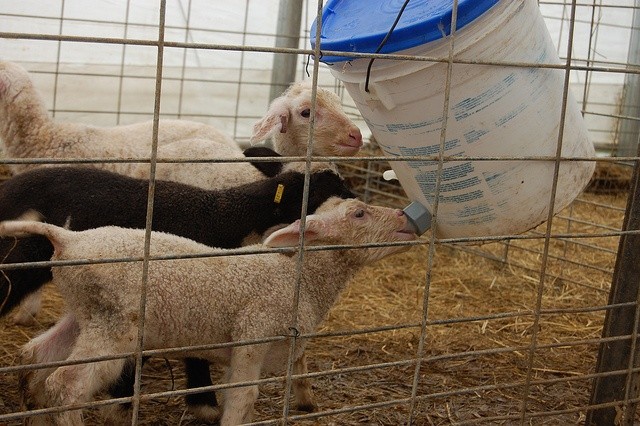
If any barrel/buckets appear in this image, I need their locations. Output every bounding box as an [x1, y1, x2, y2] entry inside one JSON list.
[[310, 1, 599, 248]]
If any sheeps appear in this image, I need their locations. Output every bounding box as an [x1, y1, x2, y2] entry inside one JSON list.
[[132, 137, 347, 410], [250, 81, 364, 162], [0, 55, 242, 326], [0, 194, 415, 426], [0, 164, 359, 426]]
